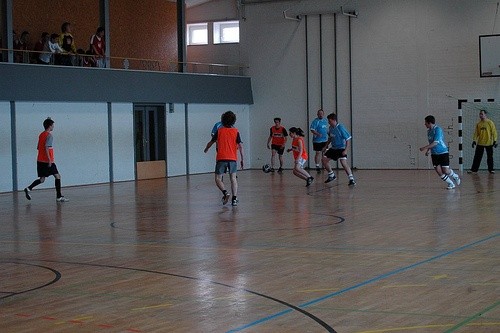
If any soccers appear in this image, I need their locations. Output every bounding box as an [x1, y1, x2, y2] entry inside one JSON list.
[[263, 163, 272, 174]]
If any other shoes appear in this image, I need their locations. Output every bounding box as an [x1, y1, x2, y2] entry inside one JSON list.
[[489, 170, 496, 174], [467, 170, 478, 174]]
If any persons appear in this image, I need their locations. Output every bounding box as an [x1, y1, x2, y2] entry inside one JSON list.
[[286, 127, 314, 187], [12, 22, 106, 68], [211, 114, 238, 182], [467, 110, 498, 174], [321, 113, 356, 186], [310, 109, 330, 171], [24, 118, 70, 202], [204, 111, 245, 207], [267, 118, 288, 175], [420, 115, 461, 190]]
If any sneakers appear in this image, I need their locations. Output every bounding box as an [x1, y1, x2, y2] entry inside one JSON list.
[[231, 198, 240, 206], [222, 192, 230, 205], [453, 174, 461, 185], [324, 175, 336, 184], [271, 168, 275, 171], [306, 177, 314, 187], [56, 197, 69, 202], [348, 180, 356, 185], [278, 167, 283, 174], [24, 188, 31, 200], [446, 183, 456, 190], [316, 167, 321, 171]]
